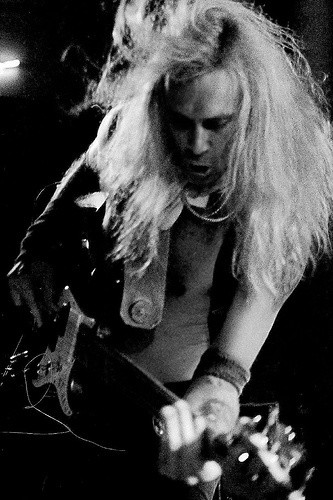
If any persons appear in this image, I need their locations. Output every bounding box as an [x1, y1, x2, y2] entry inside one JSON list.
[[7, 0, 333, 500]]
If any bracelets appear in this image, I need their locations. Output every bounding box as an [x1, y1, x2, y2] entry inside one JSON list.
[[188, 347, 250, 396]]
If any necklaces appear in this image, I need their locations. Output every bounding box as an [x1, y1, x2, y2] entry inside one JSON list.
[[181, 188, 235, 222]]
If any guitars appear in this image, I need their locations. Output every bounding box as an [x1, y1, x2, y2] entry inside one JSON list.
[[0, 236, 315, 500]]
